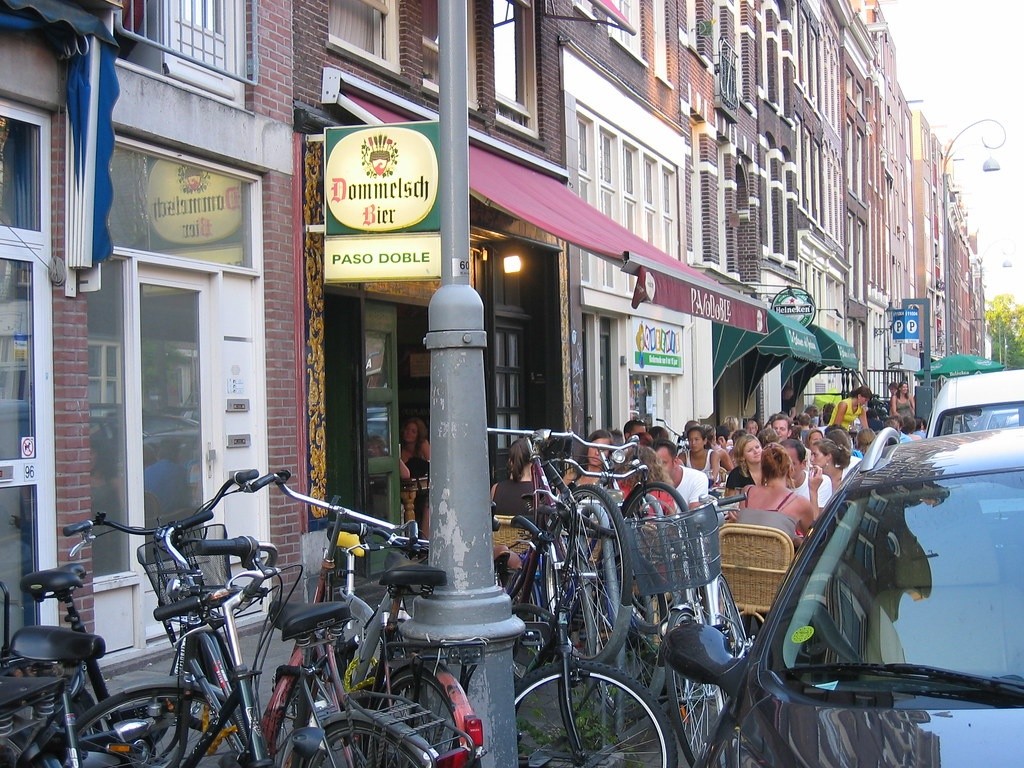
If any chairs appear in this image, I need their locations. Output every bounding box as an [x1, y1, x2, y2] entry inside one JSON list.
[[492, 513, 661, 658], [860, 490, 1024, 675], [720, 522, 795, 622]]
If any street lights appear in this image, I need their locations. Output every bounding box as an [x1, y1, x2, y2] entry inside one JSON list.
[[942, 118, 1007, 358], [978, 241, 1013, 358]]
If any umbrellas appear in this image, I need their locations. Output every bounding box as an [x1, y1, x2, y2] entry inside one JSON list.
[[914, 355, 1006, 382]]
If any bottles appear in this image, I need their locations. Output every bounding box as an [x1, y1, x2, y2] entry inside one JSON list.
[[854, 417, 861, 433]]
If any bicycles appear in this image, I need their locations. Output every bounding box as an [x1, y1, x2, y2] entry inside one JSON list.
[[0, 428, 756, 768]]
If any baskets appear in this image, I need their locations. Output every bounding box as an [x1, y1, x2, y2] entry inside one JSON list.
[[136, 524, 232, 606], [624, 502, 720, 593]]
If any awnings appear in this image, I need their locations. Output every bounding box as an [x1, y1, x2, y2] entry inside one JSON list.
[[712, 310, 822, 410], [336, 92, 769, 335], [0, 0, 120, 266], [546, 0, 636, 35], [781, 324, 857, 408]]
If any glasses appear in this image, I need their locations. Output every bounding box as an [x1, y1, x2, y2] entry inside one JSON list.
[[630, 418, 644, 427]]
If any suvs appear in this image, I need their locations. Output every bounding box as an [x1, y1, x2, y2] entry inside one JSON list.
[[668, 423, 1024, 768]]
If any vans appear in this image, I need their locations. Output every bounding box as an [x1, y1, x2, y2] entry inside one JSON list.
[[926, 365, 1024, 440]]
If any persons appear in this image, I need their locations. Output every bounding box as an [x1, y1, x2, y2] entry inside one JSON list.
[[400, 419, 430, 540], [490, 381, 927, 700], [368, 435, 389, 516], [89, 435, 196, 572]]
